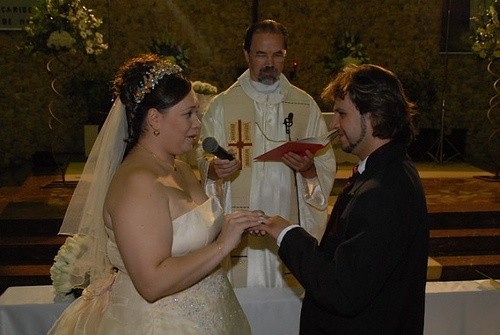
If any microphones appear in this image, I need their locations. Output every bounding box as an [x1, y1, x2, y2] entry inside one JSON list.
[[202, 136, 235, 161], [288, 113, 294, 121]]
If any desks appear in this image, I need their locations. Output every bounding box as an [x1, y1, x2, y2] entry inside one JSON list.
[[0, 279, 500, 335]]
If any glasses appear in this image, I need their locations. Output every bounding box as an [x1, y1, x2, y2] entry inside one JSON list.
[[249, 46, 287, 64]]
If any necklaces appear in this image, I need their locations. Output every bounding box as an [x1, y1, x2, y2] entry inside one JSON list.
[[135, 142, 178, 173]]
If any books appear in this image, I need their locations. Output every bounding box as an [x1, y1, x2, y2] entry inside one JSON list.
[[253, 129, 339, 162]]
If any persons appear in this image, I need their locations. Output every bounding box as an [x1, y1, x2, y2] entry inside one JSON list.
[[245, 62, 430, 335], [45, 51, 266, 335], [195, 19, 337, 289]]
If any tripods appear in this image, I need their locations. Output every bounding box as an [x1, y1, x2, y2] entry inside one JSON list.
[[411, 0, 471, 168]]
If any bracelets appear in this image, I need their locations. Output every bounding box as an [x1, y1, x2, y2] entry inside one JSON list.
[[213, 241, 224, 257]]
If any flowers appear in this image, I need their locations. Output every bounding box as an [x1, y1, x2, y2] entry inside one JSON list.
[[191, 81, 218, 98], [49, 233, 91, 296], [324, 35, 370, 72], [468, 0, 500, 61], [22, 0, 108, 59]]
